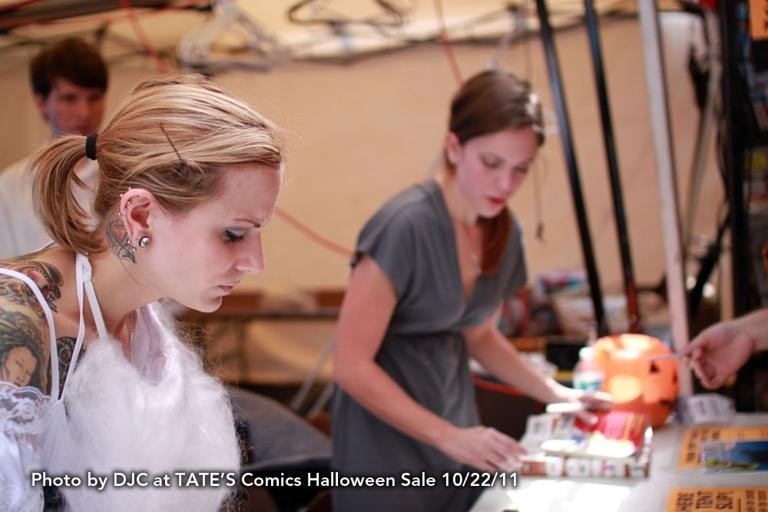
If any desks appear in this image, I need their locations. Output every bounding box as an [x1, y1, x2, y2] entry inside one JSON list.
[[473, 404, 768, 512], [175, 288, 342, 429]]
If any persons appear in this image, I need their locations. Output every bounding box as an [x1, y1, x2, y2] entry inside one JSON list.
[[0, 73, 281, 512], [0, 35, 109, 259], [332, 69, 613, 512], [683, 309, 768, 389]]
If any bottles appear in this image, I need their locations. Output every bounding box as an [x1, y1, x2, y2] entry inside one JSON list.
[[572, 347, 606, 390]]
